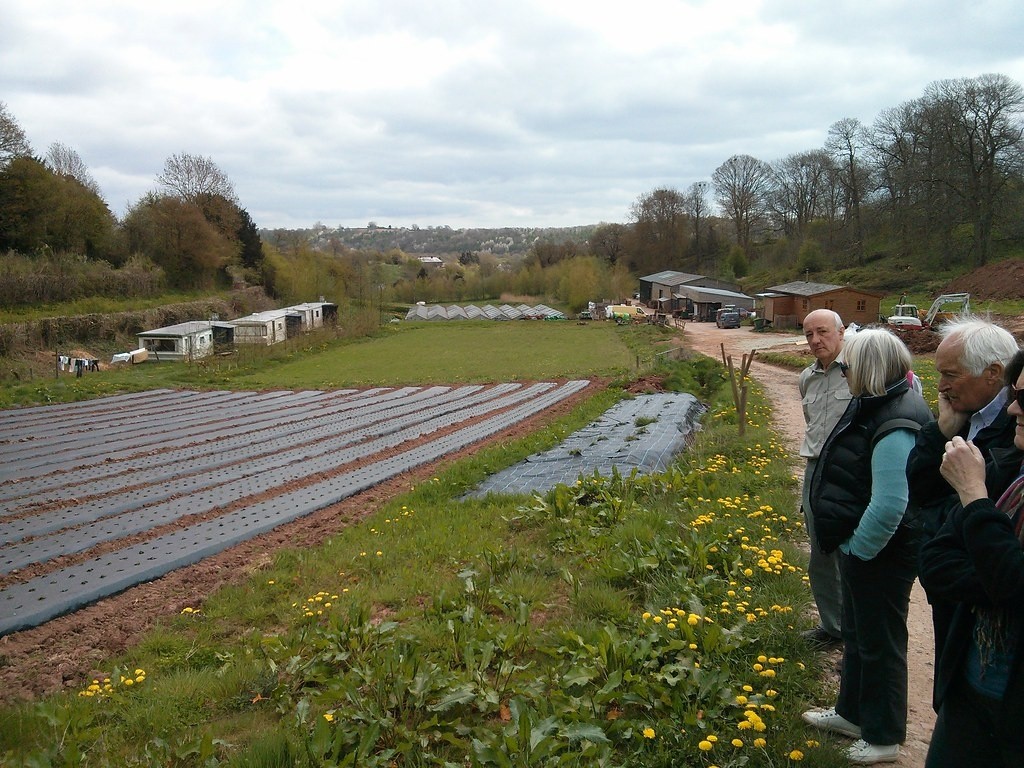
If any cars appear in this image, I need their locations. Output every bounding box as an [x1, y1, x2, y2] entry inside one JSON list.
[[632, 293, 641, 299]]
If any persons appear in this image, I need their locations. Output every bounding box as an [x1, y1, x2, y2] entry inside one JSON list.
[[919, 350, 1024, 767], [906, 305, 1024, 717], [799, 309, 854, 651], [802, 326, 936, 763]]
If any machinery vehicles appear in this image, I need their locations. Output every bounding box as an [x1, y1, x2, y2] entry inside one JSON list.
[[886, 293, 971, 332]]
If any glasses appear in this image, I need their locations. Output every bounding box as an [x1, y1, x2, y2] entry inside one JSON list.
[[1010, 382, 1024, 410], [840, 362, 852, 377]]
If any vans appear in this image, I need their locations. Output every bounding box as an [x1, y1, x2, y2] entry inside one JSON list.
[[717, 313, 740, 329], [579, 312, 591, 319]]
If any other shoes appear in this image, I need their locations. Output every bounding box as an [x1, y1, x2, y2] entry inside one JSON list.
[[836, 656, 844, 670], [800, 627, 838, 643]]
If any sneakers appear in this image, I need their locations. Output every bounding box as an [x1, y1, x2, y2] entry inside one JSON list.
[[801, 706, 863, 740], [838, 735, 901, 765]]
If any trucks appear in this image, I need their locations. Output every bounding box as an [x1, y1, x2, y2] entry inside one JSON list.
[[612, 306, 647, 318]]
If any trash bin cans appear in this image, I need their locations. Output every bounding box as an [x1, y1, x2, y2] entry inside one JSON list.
[[753, 318, 764, 332], [681, 312, 688, 317]]
